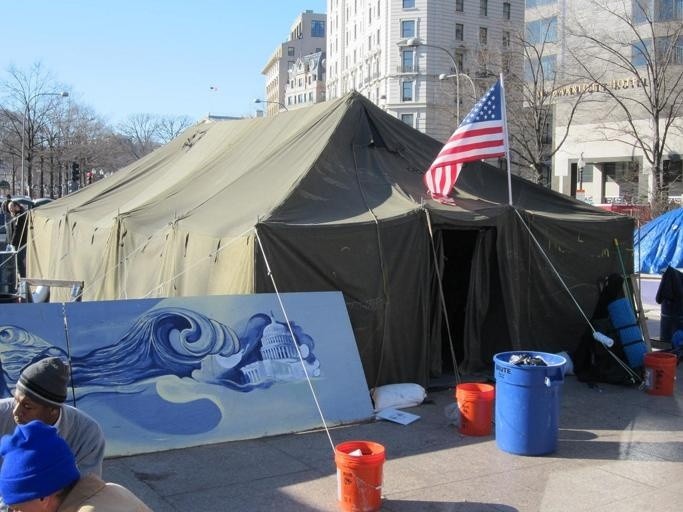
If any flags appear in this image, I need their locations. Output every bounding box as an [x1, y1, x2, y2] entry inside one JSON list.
[[421, 78, 506, 208]]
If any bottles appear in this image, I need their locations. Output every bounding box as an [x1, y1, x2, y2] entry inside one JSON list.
[[1, 266, 9, 293], [591, 331, 613, 348]]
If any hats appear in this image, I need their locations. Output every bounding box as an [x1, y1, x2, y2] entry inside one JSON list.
[[0, 419, 80, 505], [15, 357, 69, 407]]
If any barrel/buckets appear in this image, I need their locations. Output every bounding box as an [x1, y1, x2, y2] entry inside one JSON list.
[[494, 350, 565, 457], [334, 441, 386, 511], [456, 382, 494, 437], [645, 351, 678, 396]]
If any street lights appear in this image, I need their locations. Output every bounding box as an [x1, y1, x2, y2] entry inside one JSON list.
[[407, 36, 461, 128], [437, 73, 477, 106], [22, 92, 69, 197], [254, 98, 287, 111]]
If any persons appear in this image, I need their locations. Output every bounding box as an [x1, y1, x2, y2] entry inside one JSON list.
[[0, 354, 106, 481], [6, 200, 27, 278], [0, 194, 13, 244], [0, 420, 155, 511]]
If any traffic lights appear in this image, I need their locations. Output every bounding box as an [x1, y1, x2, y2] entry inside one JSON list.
[[72, 163, 79, 182]]
